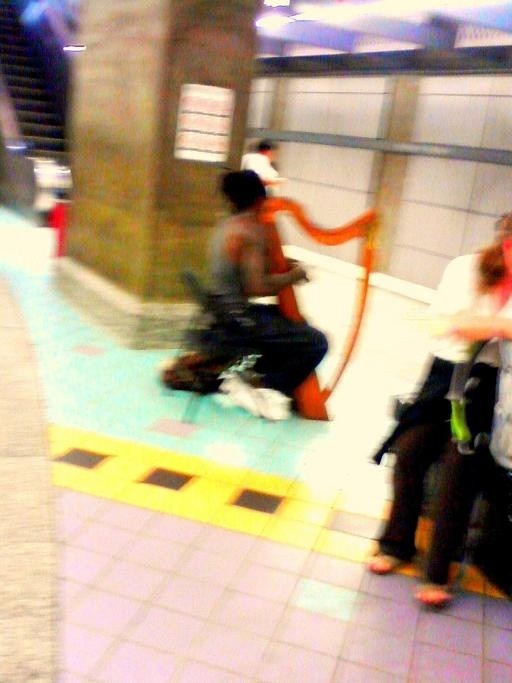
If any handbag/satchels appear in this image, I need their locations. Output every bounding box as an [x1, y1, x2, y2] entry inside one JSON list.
[[450, 338, 512, 470], [162, 352, 239, 383]]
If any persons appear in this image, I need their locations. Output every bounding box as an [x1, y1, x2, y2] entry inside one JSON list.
[[207, 168, 330, 423], [361, 211, 510, 604], [239, 137, 288, 197]]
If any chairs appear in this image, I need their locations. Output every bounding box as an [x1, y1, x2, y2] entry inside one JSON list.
[[173, 266, 291, 425]]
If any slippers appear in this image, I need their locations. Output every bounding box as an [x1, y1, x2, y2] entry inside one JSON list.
[[372, 555, 399, 573], [417, 583, 448, 605]]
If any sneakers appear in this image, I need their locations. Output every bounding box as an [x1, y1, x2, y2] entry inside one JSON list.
[[221, 377, 259, 417]]
